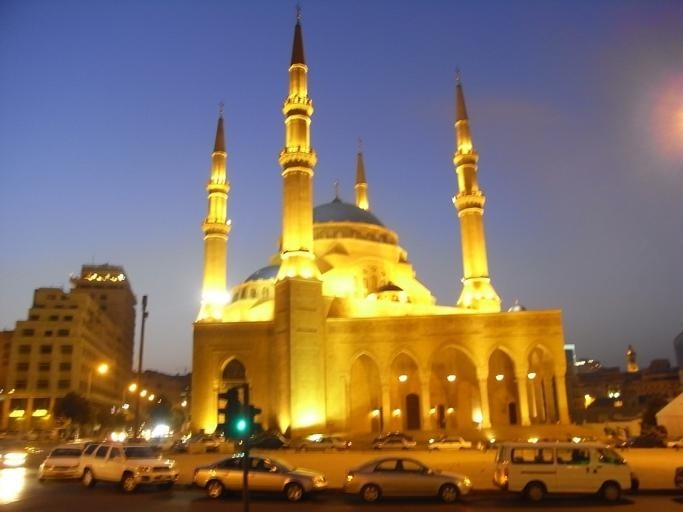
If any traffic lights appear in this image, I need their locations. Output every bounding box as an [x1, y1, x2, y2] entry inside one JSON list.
[[253, 406, 264, 434], [215, 391, 228, 434], [233, 417, 248, 435]]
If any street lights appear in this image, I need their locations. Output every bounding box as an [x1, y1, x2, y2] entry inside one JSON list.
[[120, 383, 155, 418], [134, 294, 148, 439], [87, 363, 108, 399]]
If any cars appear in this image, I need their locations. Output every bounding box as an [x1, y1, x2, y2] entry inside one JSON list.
[[342, 457, 470, 501], [616, 434, 664, 448], [108, 425, 170, 439], [369, 431, 417, 449], [428, 434, 472, 451], [237, 431, 289, 449], [674, 464, 682, 489], [295, 433, 351, 452], [37, 445, 86, 480], [0, 437, 26, 467], [667, 437, 683, 447], [172, 433, 218, 452], [193, 454, 326, 501]]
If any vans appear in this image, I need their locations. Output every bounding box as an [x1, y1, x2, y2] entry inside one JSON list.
[[491, 439, 640, 503]]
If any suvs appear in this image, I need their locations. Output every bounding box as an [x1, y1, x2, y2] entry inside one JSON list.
[[79, 440, 179, 492]]
